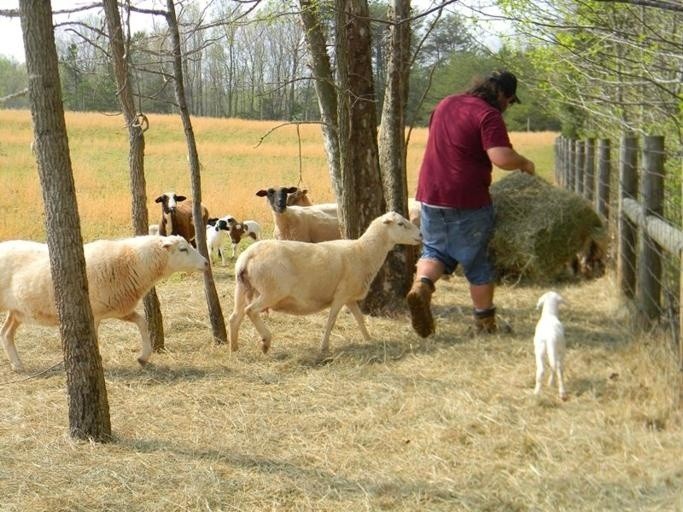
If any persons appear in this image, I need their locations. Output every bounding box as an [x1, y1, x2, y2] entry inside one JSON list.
[[404, 67, 537, 339]]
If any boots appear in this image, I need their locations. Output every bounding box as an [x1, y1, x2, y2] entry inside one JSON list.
[[473, 305, 511, 336], [407, 276, 435, 338]]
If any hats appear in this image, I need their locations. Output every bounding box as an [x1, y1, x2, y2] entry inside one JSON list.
[[488, 70, 521, 104]]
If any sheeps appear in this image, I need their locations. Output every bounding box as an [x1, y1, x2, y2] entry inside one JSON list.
[[153, 190, 209, 244], [28, 233, 210, 368], [532, 290, 568, 401], [287, 188, 313, 207], [256, 186, 341, 243], [148, 222, 162, 236], [223, 214, 262, 258], [226, 211, 424, 354], [206, 215, 228, 263], [408, 195, 422, 224]]
